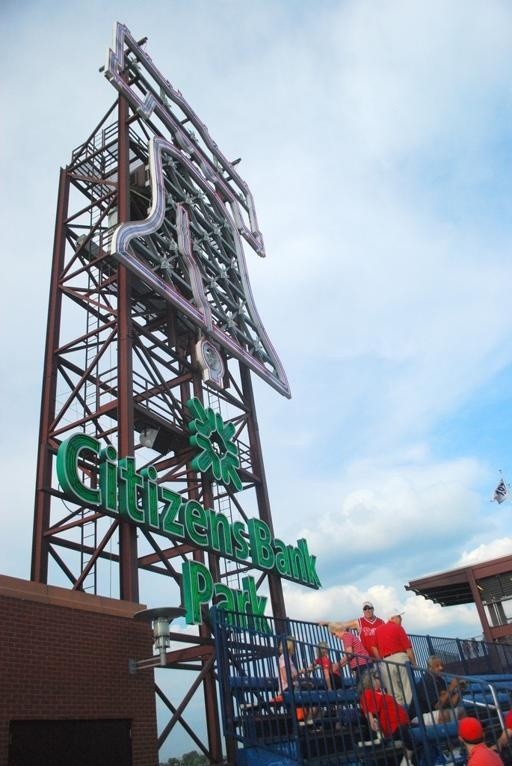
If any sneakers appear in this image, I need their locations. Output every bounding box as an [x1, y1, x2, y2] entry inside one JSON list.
[[306, 722, 349, 736], [358, 735, 380, 747], [437, 745, 465, 766]]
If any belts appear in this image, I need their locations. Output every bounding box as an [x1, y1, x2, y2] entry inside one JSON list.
[[383, 650, 407, 658]]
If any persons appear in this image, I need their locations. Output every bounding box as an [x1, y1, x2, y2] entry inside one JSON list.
[[278, 601, 512, 766]]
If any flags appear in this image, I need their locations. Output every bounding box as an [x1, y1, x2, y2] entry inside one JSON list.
[[490, 478, 510, 503]]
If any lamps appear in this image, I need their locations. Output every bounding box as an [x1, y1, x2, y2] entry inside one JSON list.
[[129, 606, 190, 674]]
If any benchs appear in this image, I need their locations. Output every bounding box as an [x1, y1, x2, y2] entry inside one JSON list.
[[228, 674, 511, 766]]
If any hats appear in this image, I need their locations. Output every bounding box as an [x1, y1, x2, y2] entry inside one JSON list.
[[386, 607, 405, 618], [362, 602, 373, 609], [459, 717, 483, 740]]
